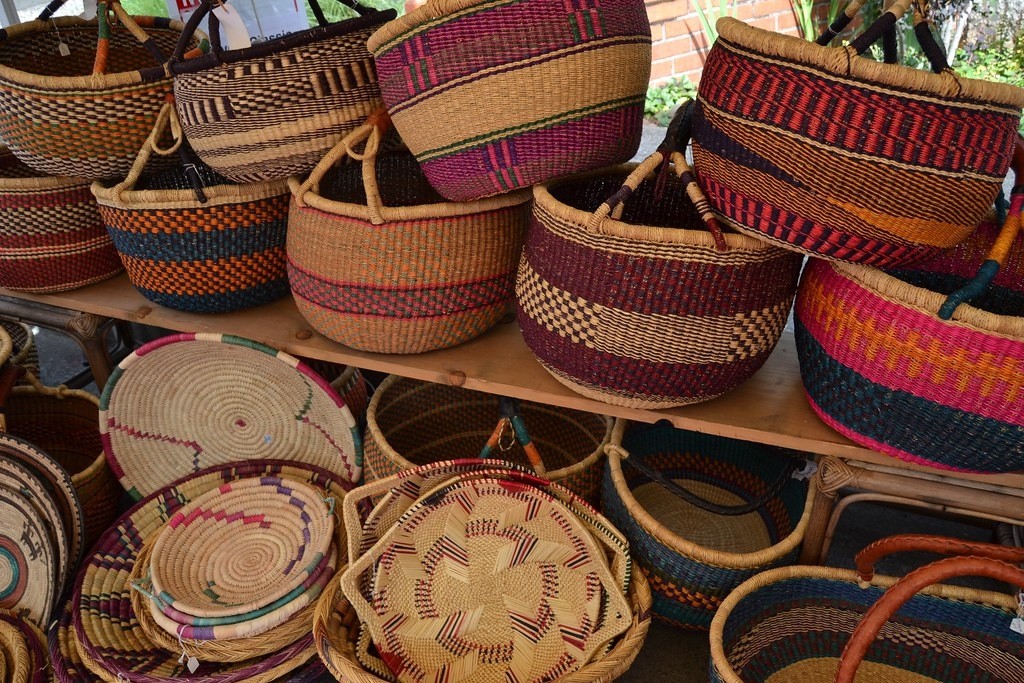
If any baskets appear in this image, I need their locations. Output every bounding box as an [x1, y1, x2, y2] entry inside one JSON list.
[[91, 90, 307, 313], [0, 319, 41, 385], [516, 99, 804, 410], [706, 533, 1024, 683], [286, 108, 533, 354], [366, 0, 652, 203], [795, 135, 1024, 476], [603, 418, 821, 633], [291, 354, 367, 439], [363, 376, 614, 508], [171, 0, 403, 184], [0, 143, 126, 294], [692, 0, 1024, 270], [0, 0, 210, 179], [0, 369, 127, 560]]
